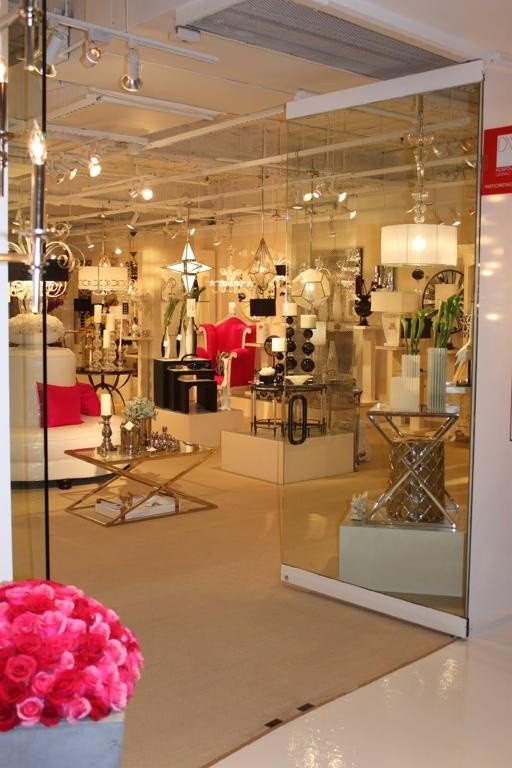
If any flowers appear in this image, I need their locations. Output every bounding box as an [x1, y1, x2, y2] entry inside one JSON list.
[[8, 312, 65, 336], [0, 579, 143, 733]]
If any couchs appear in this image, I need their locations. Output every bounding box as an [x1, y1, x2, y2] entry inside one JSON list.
[[10, 347, 132, 490], [195, 309, 268, 410]]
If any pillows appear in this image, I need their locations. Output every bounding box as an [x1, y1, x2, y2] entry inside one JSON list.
[[37, 382, 85, 429], [76, 378, 101, 417]]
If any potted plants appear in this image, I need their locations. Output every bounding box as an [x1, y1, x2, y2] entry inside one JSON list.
[[427, 292, 464, 413], [176, 286, 207, 357], [122, 396, 158, 448], [401, 306, 434, 411], [161, 296, 182, 358]]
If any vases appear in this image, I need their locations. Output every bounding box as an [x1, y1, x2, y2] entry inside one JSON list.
[[1, 709, 126, 767], [9, 333, 61, 346]]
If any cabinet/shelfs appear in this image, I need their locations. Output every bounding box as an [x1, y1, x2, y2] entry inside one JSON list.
[[153, 356, 217, 414], [310, 322, 380, 407]]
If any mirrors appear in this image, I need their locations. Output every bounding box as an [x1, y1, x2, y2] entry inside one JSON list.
[[310, 245, 364, 323]]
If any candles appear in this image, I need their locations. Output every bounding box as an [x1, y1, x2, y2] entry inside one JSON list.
[[272, 337, 287, 351], [107, 313, 115, 331], [229, 302, 236, 314], [100, 392, 112, 416], [103, 329, 110, 348], [93, 303, 103, 323], [282, 303, 297, 316], [299, 314, 317, 328]]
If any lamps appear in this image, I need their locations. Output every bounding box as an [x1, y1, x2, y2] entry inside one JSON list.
[[87, 140, 107, 164], [32, 19, 67, 79], [0, 1, 85, 315], [85, 236, 94, 249], [126, 229, 139, 280], [79, 28, 112, 70], [125, 212, 139, 229], [43, 162, 65, 185], [289, 171, 332, 311], [380, 96, 459, 268], [161, 226, 177, 240], [121, 40, 146, 91], [51, 156, 77, 181], [247, 167, 278, 293], [72, 155, 103, 178], [341, 249, 361, 302], [174, 205, 184, 224], [161, 207, 213, 296], [370, 269, 418, 312], [129, 190, 137, 199], [207, 216, 252, 295], [78, 220, 128, 290]]
